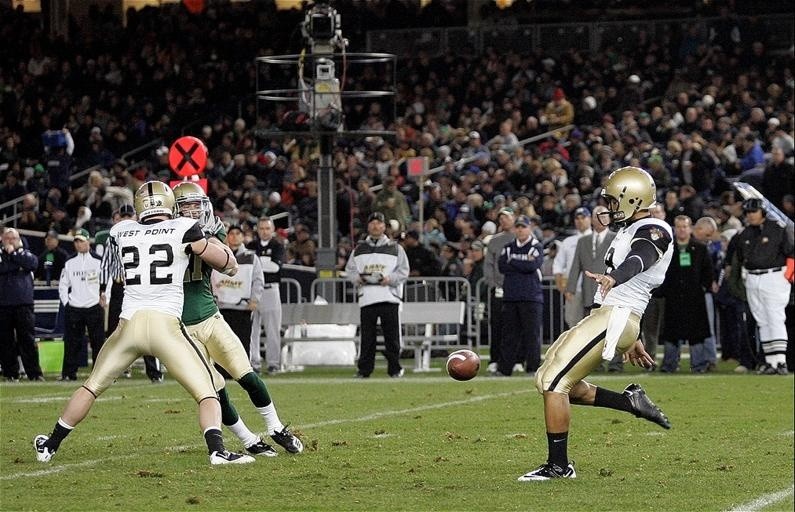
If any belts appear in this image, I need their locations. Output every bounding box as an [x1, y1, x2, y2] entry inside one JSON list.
[[747, 267, 782, 275]]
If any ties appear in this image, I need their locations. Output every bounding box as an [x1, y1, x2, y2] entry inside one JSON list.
[[595, 235, 600, 258]]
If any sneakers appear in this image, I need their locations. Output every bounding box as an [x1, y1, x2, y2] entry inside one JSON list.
[[33, 434, 52, 463], [518, 460, 577, 482], [270, 430, 304, 454], [6, 365, 277, 382], [622, 383, 672, 430], [209, 450, 256, 465], [245, 440, 279, 457]]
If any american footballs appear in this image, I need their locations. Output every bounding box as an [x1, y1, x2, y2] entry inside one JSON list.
[[445, 349, 480, 381]]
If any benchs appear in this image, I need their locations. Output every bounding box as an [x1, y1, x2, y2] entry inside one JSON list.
[[277, 300, 465, 374]]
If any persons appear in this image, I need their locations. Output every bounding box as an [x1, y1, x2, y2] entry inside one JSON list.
[[172, 181, 304, 458], [32, 181, 256, 465], [1, 0, 795, 381], [516, 166, 674, 482]]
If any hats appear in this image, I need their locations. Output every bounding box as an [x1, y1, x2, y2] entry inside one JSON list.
[[119, 204, 135, 218], [496, 207, 514, 218], [45, 229, 58, 238], [575, 207, 591, 216], [72, 227, 89, 241], [367, 211, 385, 222], [227, 224, 245, 235], [514, 214, 532, 227]]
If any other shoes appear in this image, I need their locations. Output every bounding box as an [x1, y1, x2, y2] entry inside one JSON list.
[[735, 365, 749, 372], [511, 363, 526, 373], [756, 362, 777, 374], [776, 362, 787, 374], [487, 362, 499, 374]]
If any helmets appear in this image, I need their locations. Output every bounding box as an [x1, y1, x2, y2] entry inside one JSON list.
[[135, 181, 176, 222], [741, 198, 767, 218], [172, 182, 210, 227], [600, 166, 657, 222]]
